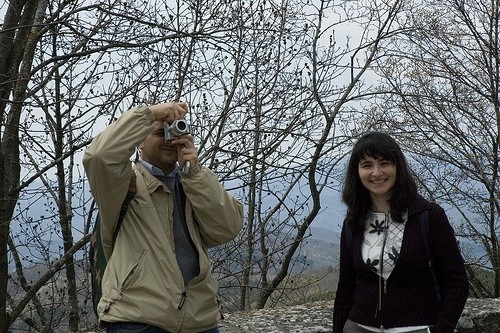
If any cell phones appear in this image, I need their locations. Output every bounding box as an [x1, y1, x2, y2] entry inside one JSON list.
[[182, 161, 191, 174]]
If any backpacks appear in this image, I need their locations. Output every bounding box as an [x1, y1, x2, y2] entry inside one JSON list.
[[89, 168, 137, 317]]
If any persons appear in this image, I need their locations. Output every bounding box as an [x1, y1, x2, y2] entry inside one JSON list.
[[332, 132, 470, 333], [81, 102, 244, 333]]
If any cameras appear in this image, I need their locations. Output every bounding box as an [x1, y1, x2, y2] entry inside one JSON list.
[[163, 120, 189, 144]]
